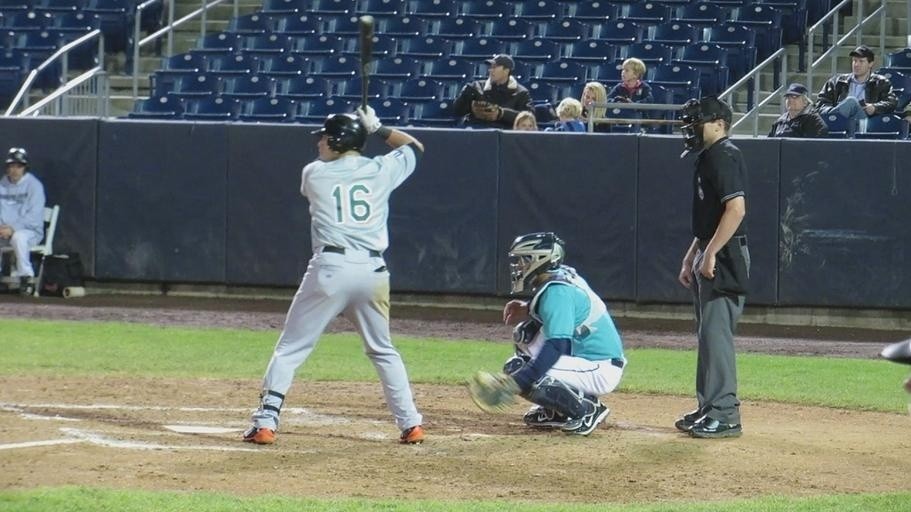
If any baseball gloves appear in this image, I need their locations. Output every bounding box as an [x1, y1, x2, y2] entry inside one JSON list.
[[466, 372, 522, 414]]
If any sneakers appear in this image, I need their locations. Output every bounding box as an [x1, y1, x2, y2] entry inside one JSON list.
[[674, 411, 703, 431], [398, 426, 425, 443], [20, 276, 36, 296], [240, 428, 276, 444], [687, 415, 742, 439], [523, 404, 567, 429], [561, 398, 610, 437]]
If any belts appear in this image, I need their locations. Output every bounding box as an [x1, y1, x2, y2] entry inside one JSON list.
[[324, 245, 380, 258], [739, 237, 746, 246]]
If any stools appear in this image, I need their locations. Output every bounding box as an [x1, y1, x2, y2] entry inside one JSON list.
[[40, 253, 85, 297]]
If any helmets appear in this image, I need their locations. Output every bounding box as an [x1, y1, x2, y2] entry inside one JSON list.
[[508, 231, 566, 297], [5, 146, 29, 165], [310, 112, 368, 152], [678, 94, 732, 159]]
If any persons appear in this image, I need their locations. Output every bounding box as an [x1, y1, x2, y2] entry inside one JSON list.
[[242, 104, 426, 445], [0, 147, 48, 295], [674, 95, 752, 439], [607, 58, 654, 134], [514, 109, 539, 132], [452, 53, 536, 128], [581, 82, 610, 128], [770, 83, 830, 137], [545, 96, 586, 132], [467, 232, 627, 437], [817, 44, 900, 117]]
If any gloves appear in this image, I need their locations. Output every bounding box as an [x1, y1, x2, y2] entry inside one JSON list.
[[358, 104, 383, 135]]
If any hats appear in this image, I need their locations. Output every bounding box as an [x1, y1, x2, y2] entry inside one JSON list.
[[848, 45, 873, 58], [484, 54, 514, 69], [783, 83, 808, 97]]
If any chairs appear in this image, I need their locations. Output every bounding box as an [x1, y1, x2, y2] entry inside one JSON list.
[[1, 198, 61, 300], [800, 45, 907, 142], [0, 1, 165, 111], [128, 0, 853, 133]]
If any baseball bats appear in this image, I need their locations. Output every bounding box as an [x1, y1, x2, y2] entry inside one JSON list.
[[360, 16, 375, 110]]
